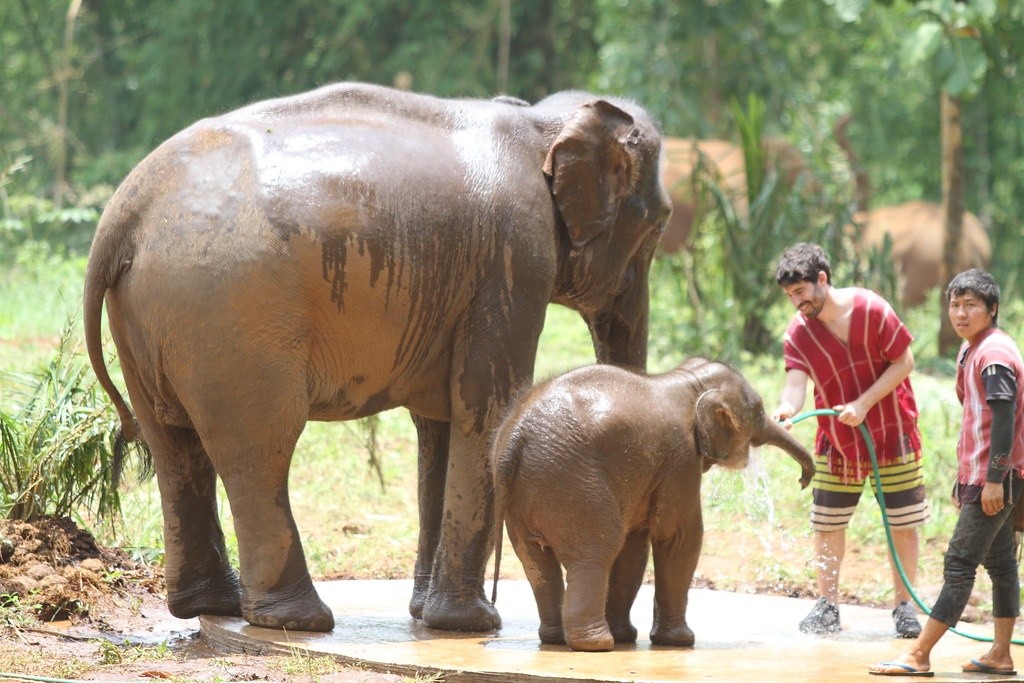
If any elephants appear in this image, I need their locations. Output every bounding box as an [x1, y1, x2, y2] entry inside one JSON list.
[[490, 359, 816, 651], [84, 82, 671, 633]]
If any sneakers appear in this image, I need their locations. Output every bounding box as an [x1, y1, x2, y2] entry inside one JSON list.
[[891, 601, 922, 638], [798, 597, 843, 637]]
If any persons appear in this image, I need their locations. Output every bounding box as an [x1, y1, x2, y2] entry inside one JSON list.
[[771, 243, 929, 638], [868, 269, 1024, 677]]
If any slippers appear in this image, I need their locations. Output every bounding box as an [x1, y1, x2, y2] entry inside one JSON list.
[[961, 657, 1018, 675], [868, 660, 935, 677]]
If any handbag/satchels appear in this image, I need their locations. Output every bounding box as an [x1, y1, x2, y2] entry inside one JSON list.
[[949, 465, 1024, 563]]
